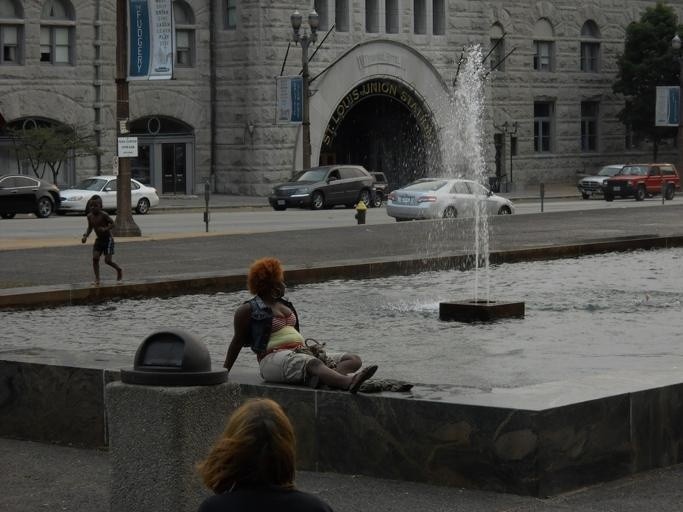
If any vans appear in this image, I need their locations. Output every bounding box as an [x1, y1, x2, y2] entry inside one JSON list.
[[268, 164, 375, 211]]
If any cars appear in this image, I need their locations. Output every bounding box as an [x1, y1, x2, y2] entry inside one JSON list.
[[55, 173, 159, 217], [0, 172, 60, 218], [386, 177, 516, 223]]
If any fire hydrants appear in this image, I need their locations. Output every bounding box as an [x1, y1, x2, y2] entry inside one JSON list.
[[352, 200, 368, 224]]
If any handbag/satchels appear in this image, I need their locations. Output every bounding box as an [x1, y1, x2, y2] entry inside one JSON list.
[[295, 337, 337, 371]]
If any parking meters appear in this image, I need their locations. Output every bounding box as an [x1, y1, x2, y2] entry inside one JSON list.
[[660, 178, 666, 203], [201, 180, 211, 234], [538, 182, 547, 214]]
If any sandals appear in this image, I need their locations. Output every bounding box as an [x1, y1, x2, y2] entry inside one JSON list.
[[349, 363, 379, 395]]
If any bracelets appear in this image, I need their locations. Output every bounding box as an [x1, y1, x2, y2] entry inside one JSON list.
[[82, 233, 87, 236]]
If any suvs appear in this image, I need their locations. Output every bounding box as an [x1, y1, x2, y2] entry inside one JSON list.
[[604, 163, 681, 202], [367, 170, 389, 209], [577, 163, 647, 201]]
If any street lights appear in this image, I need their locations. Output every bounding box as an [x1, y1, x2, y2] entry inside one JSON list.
[[671, 33, 682, 100], [285, 9, 320, 172], [501, 120, 520, 184]]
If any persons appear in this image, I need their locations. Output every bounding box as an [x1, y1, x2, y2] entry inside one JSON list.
[[223, 259, 377, 394], [81, 195, 123, 286], [193, 399, 333, 512]]
